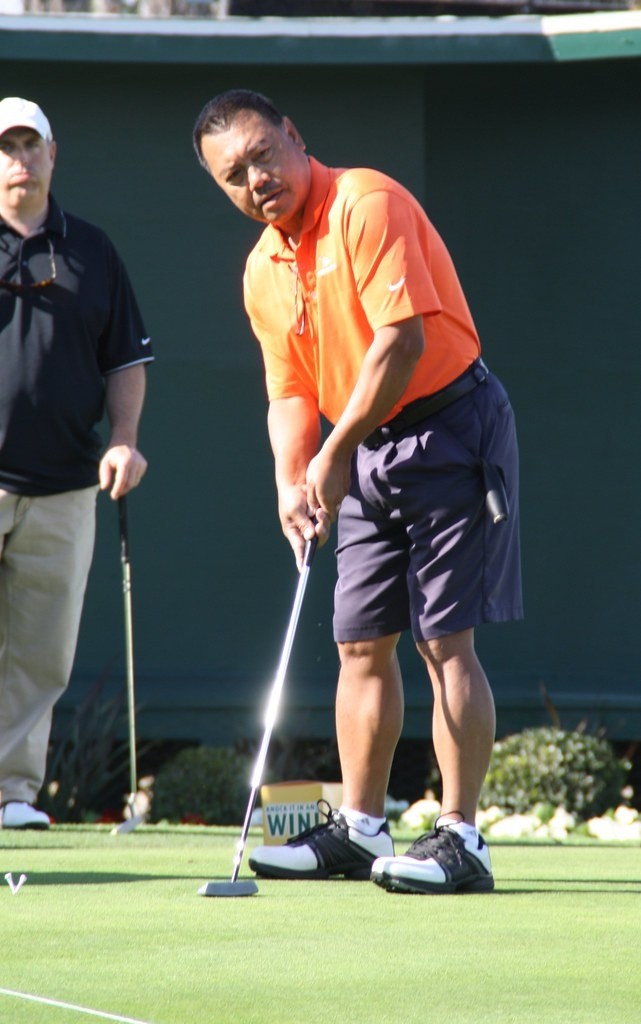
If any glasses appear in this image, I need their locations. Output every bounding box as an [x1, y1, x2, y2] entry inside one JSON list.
[[288, 261, 306, 336], [0, 240, 56, 295]]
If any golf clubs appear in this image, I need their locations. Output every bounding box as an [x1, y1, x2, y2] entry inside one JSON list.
[[111, 495, 143, 837], [196, 511, 317, 897]]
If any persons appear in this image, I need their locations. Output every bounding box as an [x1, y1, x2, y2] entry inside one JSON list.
[[0, 98, 155, 831], [191, 89, 525, 894]]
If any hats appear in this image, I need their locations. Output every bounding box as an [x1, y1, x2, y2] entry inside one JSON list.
[[0, 97, 52, 141]]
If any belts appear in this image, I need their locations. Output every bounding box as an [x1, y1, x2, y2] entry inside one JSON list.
[[386, 362, 489, 436]]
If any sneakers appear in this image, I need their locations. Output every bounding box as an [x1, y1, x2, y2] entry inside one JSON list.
[[248, 799, 395, 878], [369, 811, 495, 894], [0, 802, 50, 830]]
